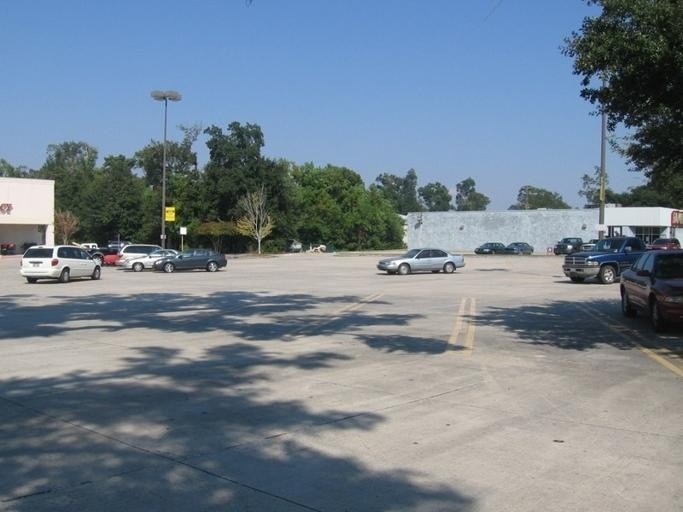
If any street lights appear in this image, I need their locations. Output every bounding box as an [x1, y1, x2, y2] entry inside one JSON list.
[[148, 88, 183, 250]]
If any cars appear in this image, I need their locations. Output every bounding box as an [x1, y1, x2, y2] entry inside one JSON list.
[[618, 248, 682, 334], [474, 232, 683, 285], [376, 249, 466, 276], [0, 239, 227, 283]]
